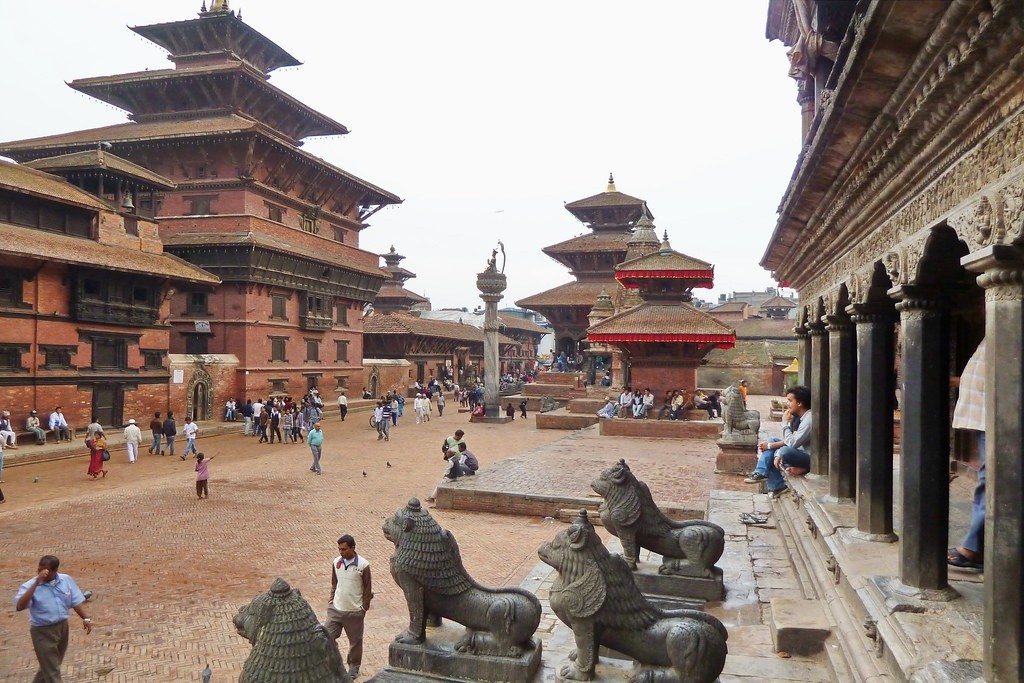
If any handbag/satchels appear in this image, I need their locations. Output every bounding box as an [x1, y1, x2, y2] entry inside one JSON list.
[[102, 450, 110, 461]]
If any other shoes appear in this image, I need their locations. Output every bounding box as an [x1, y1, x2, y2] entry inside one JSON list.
[[199, 497, 203, 499], [608, 413, 721, 421], [90, 477, 97, 480], [224, 417, 325, 445], [131, 461, 135, 464], [180, 456, 185, 461], [67, 439, 72, 442], [316, 470, 321, 475], [193, 453, 198, 457], [149, 448, 152, 454], [161, 450, 164, 456], [103, 470, 108, 477], [36, 441, 44, 445], [10, 444, 18, 448], [57, 440, 61, 444], [205, 495, 209, 499]]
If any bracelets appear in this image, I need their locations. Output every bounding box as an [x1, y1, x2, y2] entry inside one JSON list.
[[83, 618, 90, 622]]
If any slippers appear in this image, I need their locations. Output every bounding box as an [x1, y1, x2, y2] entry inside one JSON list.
[[738, 513, 768, 524]]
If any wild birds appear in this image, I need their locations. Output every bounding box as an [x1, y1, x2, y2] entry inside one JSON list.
[[362, 460, 391, 477]]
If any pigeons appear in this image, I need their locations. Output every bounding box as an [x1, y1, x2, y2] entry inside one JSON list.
[[84, 590, 92, 600], [202, 663, 211, 683]]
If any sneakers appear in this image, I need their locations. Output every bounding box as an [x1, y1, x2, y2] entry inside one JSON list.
[[425, 496, 435, 503], [348, 668, 359, 679], [743, 472, 768, 483], [769, 485, 788, 498]]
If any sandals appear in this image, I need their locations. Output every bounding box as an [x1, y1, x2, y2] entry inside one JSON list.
[[947, 548, 983, 568]]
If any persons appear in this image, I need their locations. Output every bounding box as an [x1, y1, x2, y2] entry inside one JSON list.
[[194, 451, 220, 498], [457, 442, 479, 475], [744, 408, 800, 500], [87, 431, 108, 480], [124, 418, 142, 463], [307, 423, 323, 474], [85, 416, 107, 442], [161, 410, 177, 457], [0, 435, 11, 483], [179, 417, 199, 461], [325, 534, 372, 680], [948, 333, 989, 572], [0, 487, 5, 504], [25, 409, 46, 445], [48, 407, 71, 443], [424, 449, 460, 503], [0, 410, 17, 448], [149, 412, 162, 454], [224, 349, 747, 444], [14, 555, 92, 683], [774, 386, 812, 476], [441, 429, 465, 461]]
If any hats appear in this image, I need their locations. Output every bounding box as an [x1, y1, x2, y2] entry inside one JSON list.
[[2, 411, 10, 416], [31, 409, 38, 414], [129, 419, 135, 423]]
